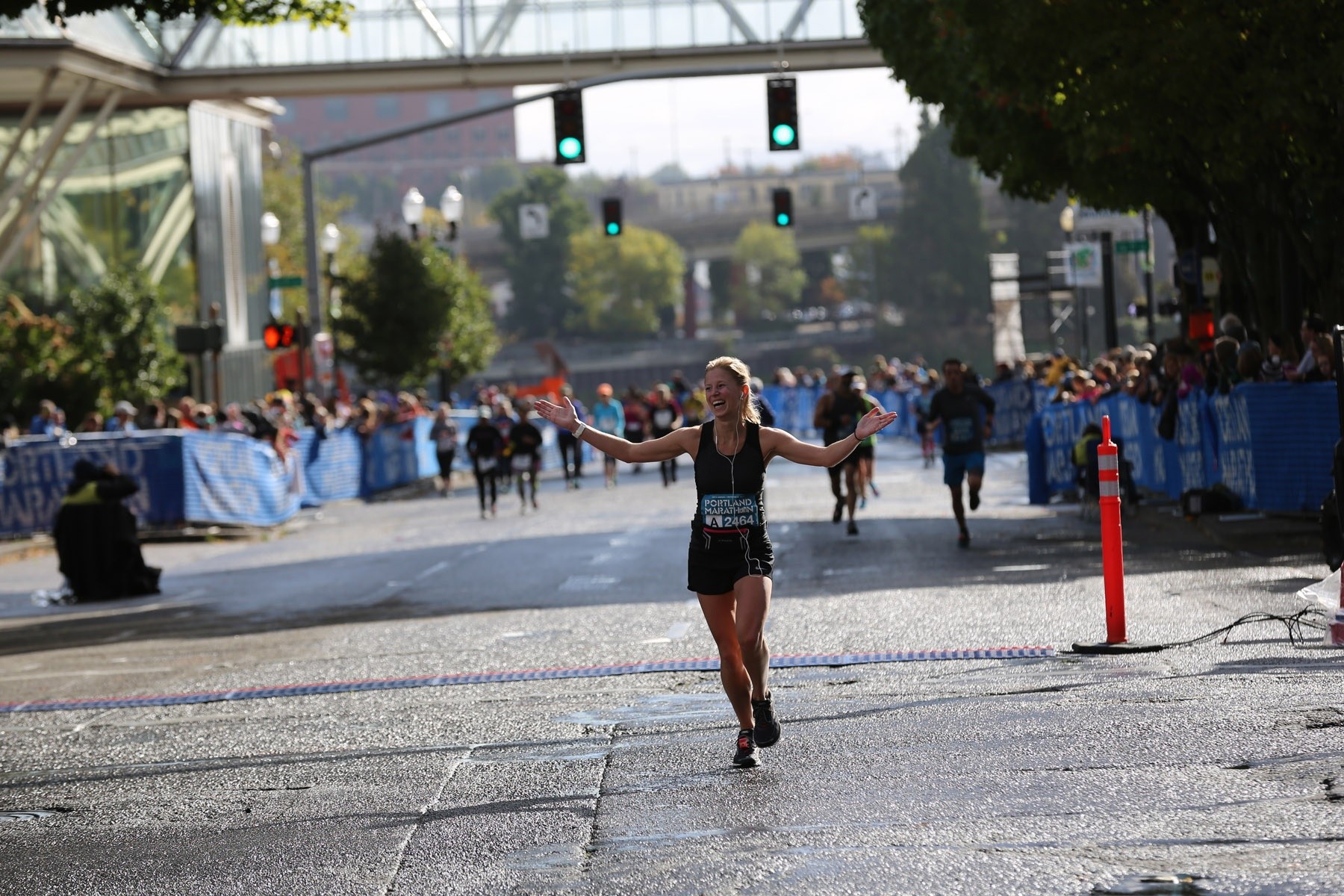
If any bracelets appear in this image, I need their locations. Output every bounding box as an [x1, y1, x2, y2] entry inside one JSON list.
[[854, 430, 864, 441], [571, 422, 587, 439]]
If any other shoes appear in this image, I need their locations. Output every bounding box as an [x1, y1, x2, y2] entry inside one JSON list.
[[832, 497, 846, 522], [968, 489, 981, 511], [958, 532, 971, 548], [846, 520, 859, 535]]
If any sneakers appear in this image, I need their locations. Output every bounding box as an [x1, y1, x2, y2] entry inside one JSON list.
[[732, 726, 760, 768], [751, 688, 781, 748]]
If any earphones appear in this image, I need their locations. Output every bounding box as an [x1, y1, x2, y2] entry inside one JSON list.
[[740, 392, 744, 399]]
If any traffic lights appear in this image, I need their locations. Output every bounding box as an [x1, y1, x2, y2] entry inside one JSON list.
[[766, 76, 800, 151], [600, 196, 623, 236], [772, 187, 795, 229], [175, 326, 213, 356], [552, 88, 588, 165], [262, 323, 300, 351]]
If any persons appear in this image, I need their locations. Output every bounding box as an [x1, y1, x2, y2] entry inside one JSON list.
[[533, 357, 898, 767], [50, 458, 159, 600], [30, 312, 1335, 536], [922, 359, 997, 549]]
